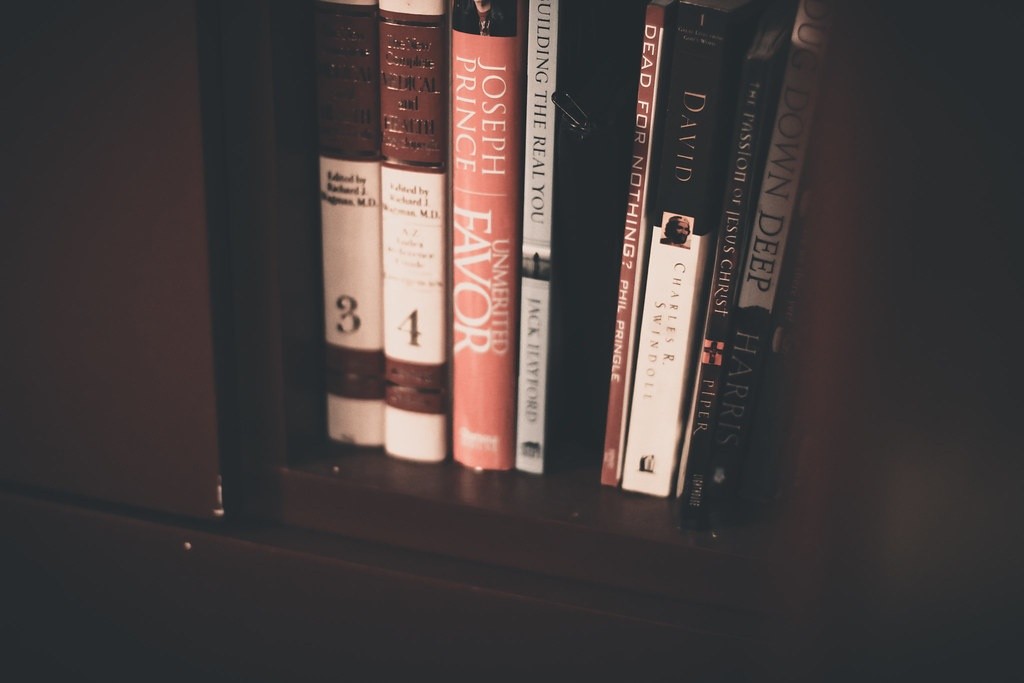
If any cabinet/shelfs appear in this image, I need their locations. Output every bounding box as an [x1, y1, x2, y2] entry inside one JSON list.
[[0, 0, 1024, 683]]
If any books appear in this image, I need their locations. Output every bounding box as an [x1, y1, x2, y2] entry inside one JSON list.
[[306, 0, 840, 531]]
